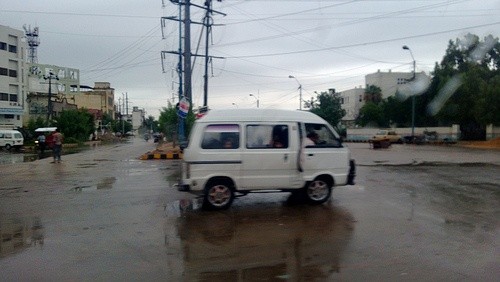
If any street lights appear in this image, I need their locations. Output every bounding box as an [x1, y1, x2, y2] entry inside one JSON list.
[[249, 94, 259, 108], [289, 76, 302, 112], [402, 45, 416, 129]]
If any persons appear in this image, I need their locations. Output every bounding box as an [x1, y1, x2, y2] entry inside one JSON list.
[[37, 132, 46, 153], [49, 128, 64, 163], [299, 132, 320, 172]]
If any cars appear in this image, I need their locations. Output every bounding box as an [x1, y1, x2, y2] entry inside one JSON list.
[[371, 130, 400, 144]]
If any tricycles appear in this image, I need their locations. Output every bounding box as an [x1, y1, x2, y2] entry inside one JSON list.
[[34, 128, 58, 152]]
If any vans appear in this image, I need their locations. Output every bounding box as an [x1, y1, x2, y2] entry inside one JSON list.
[[0, 130, 23, 149], [183, 203, 353, 270], [179, 109, 356, 209]]
